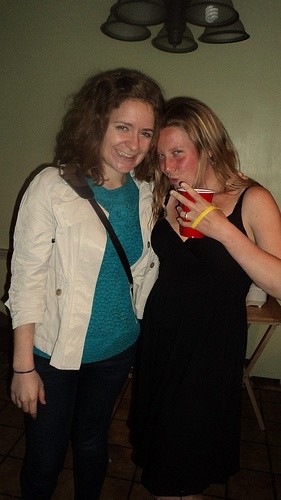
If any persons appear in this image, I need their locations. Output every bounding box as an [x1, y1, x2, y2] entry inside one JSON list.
[[126, 97, 281, 500], [3, 67, 161, 500]]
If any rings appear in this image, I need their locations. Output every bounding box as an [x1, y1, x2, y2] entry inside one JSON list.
[[185, 212, 187, 220]]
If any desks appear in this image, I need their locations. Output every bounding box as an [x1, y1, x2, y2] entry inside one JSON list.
[[243, 294, 281, 430]]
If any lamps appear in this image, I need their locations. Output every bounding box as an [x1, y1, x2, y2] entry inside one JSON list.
[[99, 1, 250, 54]]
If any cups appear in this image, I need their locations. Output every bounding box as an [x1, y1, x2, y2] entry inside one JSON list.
[[177, 188, 215, 238]]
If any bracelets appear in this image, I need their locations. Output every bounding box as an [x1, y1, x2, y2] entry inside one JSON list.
[[13, 367, 36, 374], [191, 206, 224, 228]]
